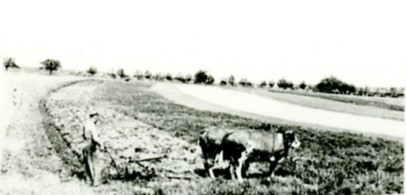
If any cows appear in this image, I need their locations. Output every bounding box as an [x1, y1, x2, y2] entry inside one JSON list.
[[197, 123, 277, 179], [222, 129, 301, 183]]
